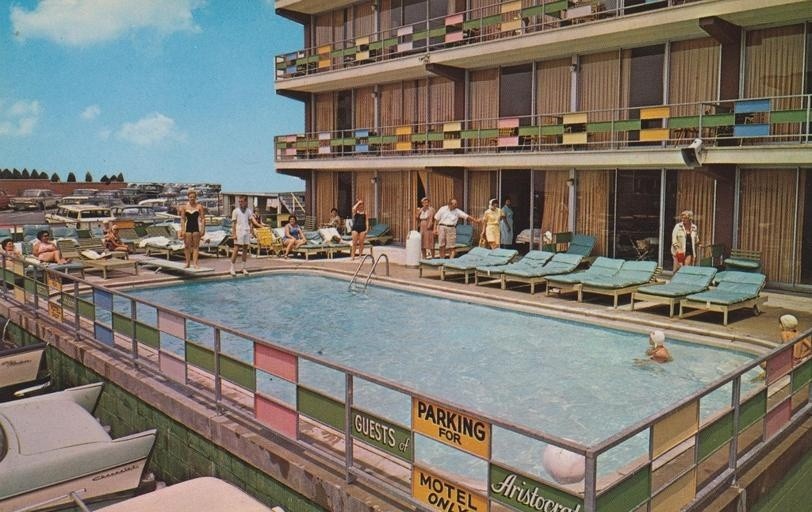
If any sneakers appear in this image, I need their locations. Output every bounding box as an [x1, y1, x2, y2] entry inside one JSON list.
[[241, 269, 249, 275], [230, 269, 236, 276]]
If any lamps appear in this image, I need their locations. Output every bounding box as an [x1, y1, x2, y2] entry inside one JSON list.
[[681, 139, 703, 167]]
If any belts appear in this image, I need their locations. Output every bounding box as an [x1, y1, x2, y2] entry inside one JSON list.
[[439, 223, 455, 228]]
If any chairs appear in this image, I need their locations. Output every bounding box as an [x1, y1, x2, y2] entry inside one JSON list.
[[679, 270, 767, 326], [0, 214, 391, 283], [555, 231, 598, 266], [632, 265, 717, 318], [543, 255, 625, 302], [579, 260, 666, 310], [723, 257, 763, 274], [441, 248, 518, 284], [420, 247, 490, 279], [503, 252, 583, 294], [567, 233, 597, 259], [474, 249, 555, 289]]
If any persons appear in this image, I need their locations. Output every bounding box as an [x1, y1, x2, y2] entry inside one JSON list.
[[751, 315, 812, 383], [351, 200, 369, 260], [416, 198, 514, 259], [671, 211, 698, 270], [103, 220, 111, 237], [329, 208, 342, 235], [230, 197, 263, 275], [105, 225, 130, 252], [647, 331, 670, 362], [32, 230, 72, 265], [250, 208, 264, 228], [2, 239, 21, 258], [282, 216, 307, 258], [180, 189, 205, 269]]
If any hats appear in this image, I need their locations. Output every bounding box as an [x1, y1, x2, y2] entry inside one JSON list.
[[649, 330, 666, 348]]
